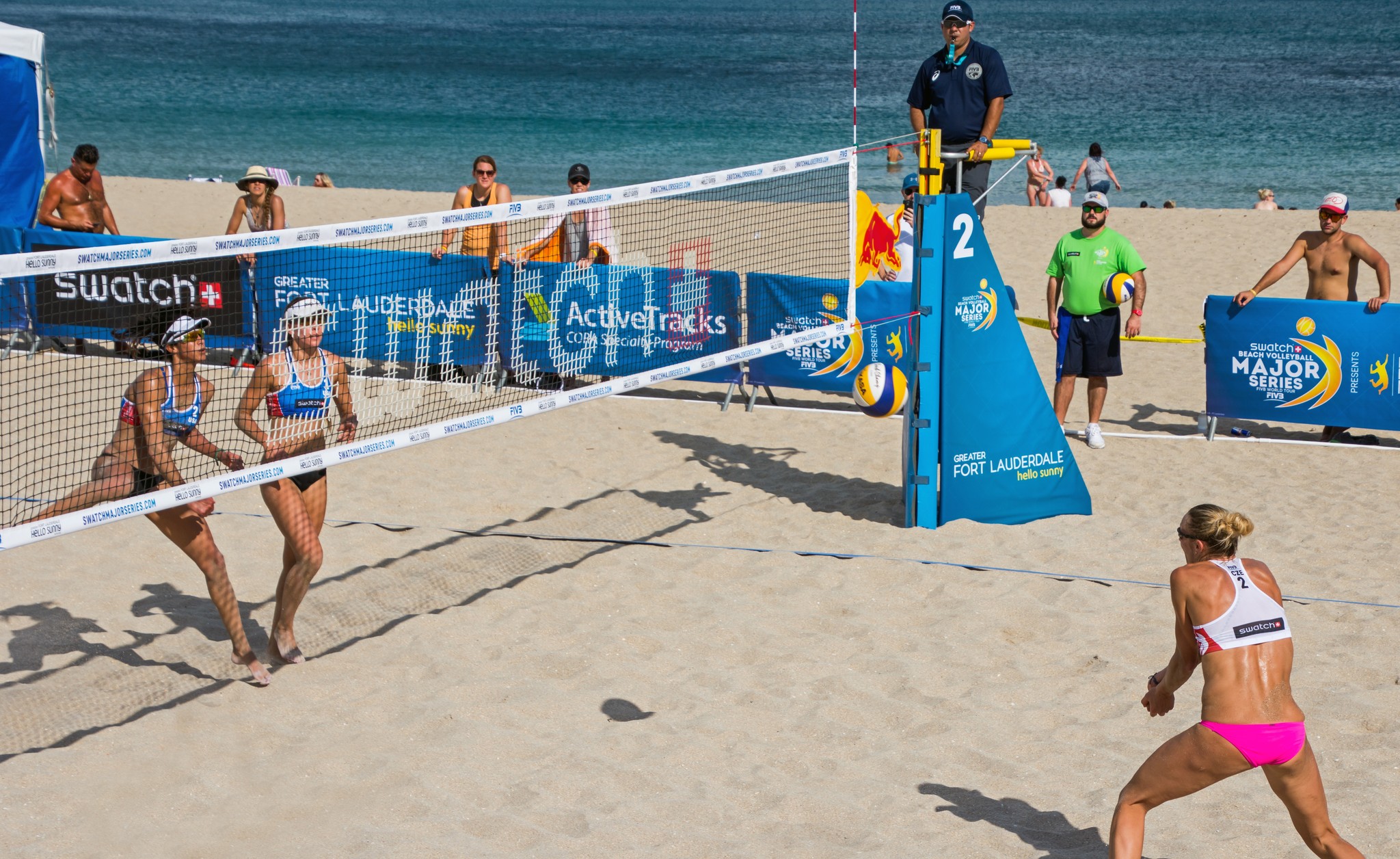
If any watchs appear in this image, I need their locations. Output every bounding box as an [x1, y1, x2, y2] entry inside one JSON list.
[[1131, 309, 1143, 316], [978, 136, 990, 145]]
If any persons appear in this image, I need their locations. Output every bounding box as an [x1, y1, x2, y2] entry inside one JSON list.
[[1026, 145, 1053, 207], [312, 172, 336, 187], [905, 1, 1014, 233], [428, 153, 512, 383], [1232, 191, 1391, 440], [35, 143, 132, 355], [1070, 142, 1121, 203], [1140, 200, 1176, 209], [885, 143, 904, 164], [13, 316, 274, 686], [1107, 503, 1371, 859], [1044, 175, 1073, 207], [1038, 189, 1146, 449], [1395, 197, 1400, 211], [872, 173, 923, 286], [1251, 188, 1299, 210], [512, 161, 612, 385], [234, 298, 358, 667], [224, 165, 293, 363]]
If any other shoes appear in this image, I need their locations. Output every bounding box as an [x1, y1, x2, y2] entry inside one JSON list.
[[1320, 425, 1351, 442]]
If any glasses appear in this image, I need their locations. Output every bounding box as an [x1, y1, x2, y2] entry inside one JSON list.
[[570, 177, 589, 185], [1177, 527, 1209, 541], [904, 188, 919, 196], [1320, 209, 1341, 221], [944, 20, 964, 28], [1082, 204, 1106, 213], [314, 177, 319, 182], [473, 169, 496, 177], [294, 316, 327, 326], [1269, 194, 1275, 197], [177, 328, 204, 343]]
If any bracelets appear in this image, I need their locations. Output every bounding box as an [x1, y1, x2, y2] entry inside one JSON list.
[[1248, 289, 1257, 296], [213, 449, 225, 465], [591, 249, 596, 258], [498, 253, 507, 260], [1148, 672, 1160, 686], [441, 246, 447, 252]]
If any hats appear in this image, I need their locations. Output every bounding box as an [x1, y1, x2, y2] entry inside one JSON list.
[[1317, 192, 1350, 215], [235, 166, 279, 194], [568, 163, 591, 181], [159, 315, 211, 353], [284, 298, 333, 321], [1081, 191, 1108, 209], [902, 172, 919, 190], [942, 0, 973, 22]]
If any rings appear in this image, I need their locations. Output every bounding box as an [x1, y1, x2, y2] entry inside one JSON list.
[[349, 433, 353, 437], [979, 153, 982, 154]]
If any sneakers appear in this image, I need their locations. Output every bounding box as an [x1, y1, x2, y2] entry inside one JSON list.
[[1085, 422, 1106, 449]]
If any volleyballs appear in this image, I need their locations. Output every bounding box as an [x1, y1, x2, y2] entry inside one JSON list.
[[852, 362, 911, 419], [1102, 271, 1135, 304]]
